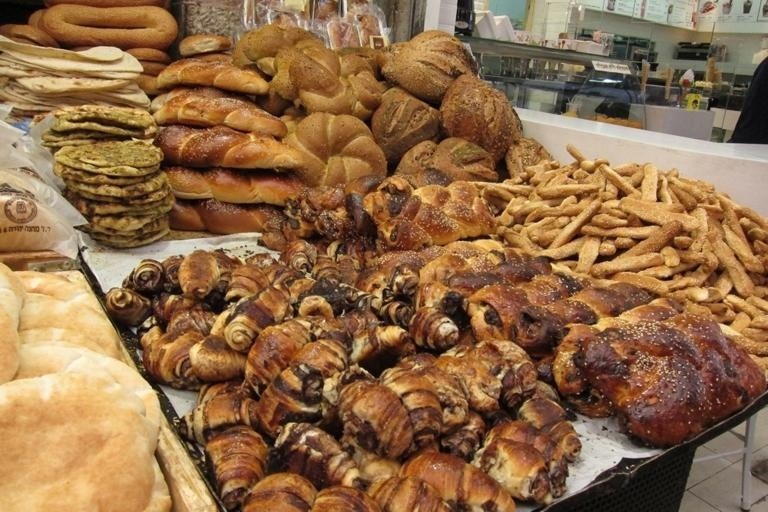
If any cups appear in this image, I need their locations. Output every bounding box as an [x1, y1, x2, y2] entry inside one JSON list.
[[722, 4, 731, 15], [743, 3, 752, 14], [565, 102, 582, 117], [680, 87, 710, 110]]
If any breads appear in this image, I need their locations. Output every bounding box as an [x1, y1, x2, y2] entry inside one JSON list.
[[105, 249, 582, 511], [482, 145, 767, 369], [1, 260, 172, 511], [1, 1, 556, 261]]
